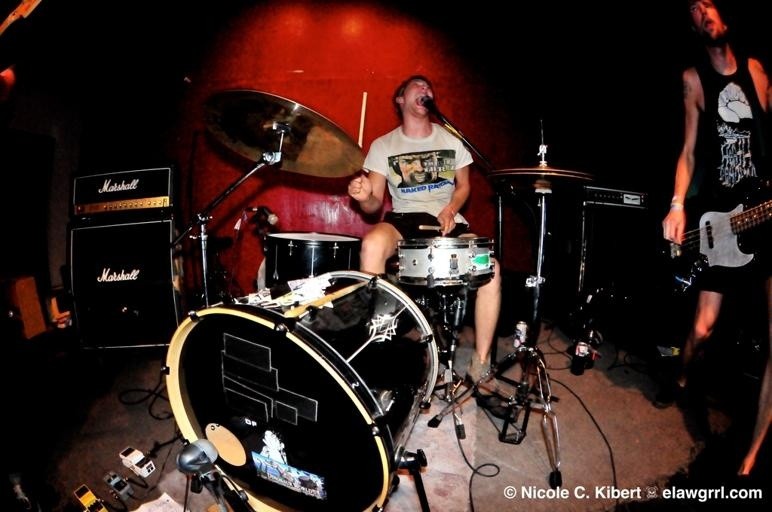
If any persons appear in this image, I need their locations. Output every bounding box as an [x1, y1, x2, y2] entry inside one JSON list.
[[392, 149, 446, 189], [658, 0, 772, 477], [346, 74, 501, 398]]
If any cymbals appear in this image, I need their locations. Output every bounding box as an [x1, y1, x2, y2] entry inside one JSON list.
[[202, 90, 365, 178], [492, 167, 592, 181]]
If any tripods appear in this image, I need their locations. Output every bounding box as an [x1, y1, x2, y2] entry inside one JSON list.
[[428, 194, 562, 491]]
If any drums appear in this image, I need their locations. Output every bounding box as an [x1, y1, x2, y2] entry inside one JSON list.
[[396, 237, 495, 288], [263, 231, 362, 289], [164, 270, 439, 512]]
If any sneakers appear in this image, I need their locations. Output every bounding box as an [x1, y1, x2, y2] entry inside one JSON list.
[[654, 382, 689, 407]]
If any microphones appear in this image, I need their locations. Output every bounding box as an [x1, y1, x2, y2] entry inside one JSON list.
[[421, 95, 446, 123], [247, 206, 278, 225], [176, 438, 220, 476]]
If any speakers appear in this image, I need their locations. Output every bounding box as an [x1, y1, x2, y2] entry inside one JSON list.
[[70, 219, 179, 350]]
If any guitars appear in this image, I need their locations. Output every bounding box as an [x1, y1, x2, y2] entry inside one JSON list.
[[670, 200, 772, 292]]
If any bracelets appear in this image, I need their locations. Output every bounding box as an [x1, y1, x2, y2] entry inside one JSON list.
[[668, 203, 684, 212]]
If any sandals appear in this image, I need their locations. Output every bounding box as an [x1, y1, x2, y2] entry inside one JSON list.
[[464, 372, 518, 420]]
